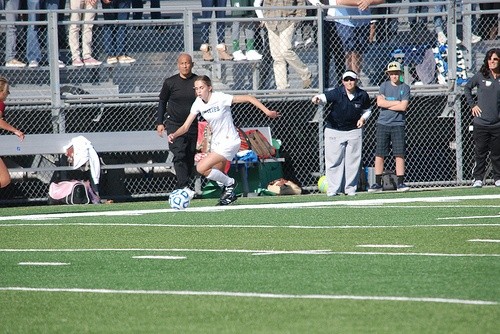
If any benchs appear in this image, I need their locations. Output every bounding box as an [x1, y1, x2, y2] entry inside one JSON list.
[[0, 63, 103, 83], [105, 59, 262, 91], [0, 126, 286, 198]]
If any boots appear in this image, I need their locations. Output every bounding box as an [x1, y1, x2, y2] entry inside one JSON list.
[[201, 44, 215, 61], [216, 44, 234, 61]]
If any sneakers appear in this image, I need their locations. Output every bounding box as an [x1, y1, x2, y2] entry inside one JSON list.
[[216, 194, 238, 206], [220, 179, 239, 200]]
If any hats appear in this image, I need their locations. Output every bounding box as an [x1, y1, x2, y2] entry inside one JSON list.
[[386, 61, 402, 71], [342, 72, 357, 79]]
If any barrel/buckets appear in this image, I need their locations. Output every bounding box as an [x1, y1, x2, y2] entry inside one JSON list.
[[364, 166, 376, 190]]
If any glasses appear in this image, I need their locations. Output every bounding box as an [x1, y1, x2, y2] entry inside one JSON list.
[[488, 57, 499, 62], [343, 77, 355, 81]]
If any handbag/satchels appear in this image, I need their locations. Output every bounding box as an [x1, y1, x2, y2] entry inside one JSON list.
[[47, 179, 93, 205], [380, 171, 398, 190], [267, 179, 301, 196]]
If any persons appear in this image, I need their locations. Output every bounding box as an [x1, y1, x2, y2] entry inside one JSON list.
[[5, 0, 161, 67], [367, 61, 410, 193], [306, 0, 402, 87], [464, 48, 500, 188], [168, 75, 281, 206], [407, 0, 481, 44], [0, 75, 24, 188], [157, 53, 200, 198], [254, 0, 313, 89], [198, 0, 265, 61], [312, 70, 371, 197]]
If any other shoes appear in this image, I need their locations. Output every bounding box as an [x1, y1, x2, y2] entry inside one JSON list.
[[119, 56, 136, 64], [58, 59, 67, 69], [472, 33, 481, 45], [245, 50, 263, 61], [73, 58, 84, 66], [5, 60, 26, 67], [438, 30, 449, 46], [107, 57, 117, 64], [455, 37, 460, 44], [83, 57, 102, 66], [494, 181, 500, 187], [302, 75, 312, 89], [473, 180, 483, 188], [232, 49, 246, 61], [27, 60, 39, 68], [368, 184, 382, 192], [397, 184, 410, 192]]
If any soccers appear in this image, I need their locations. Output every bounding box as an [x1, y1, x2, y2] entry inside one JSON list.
[[168, 189, 190, 210], [317, 176, 327, 193]]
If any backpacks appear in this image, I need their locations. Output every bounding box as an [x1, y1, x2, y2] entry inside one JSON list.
[[234, 150, 258, 166], [244, 129, 277, 161]]
